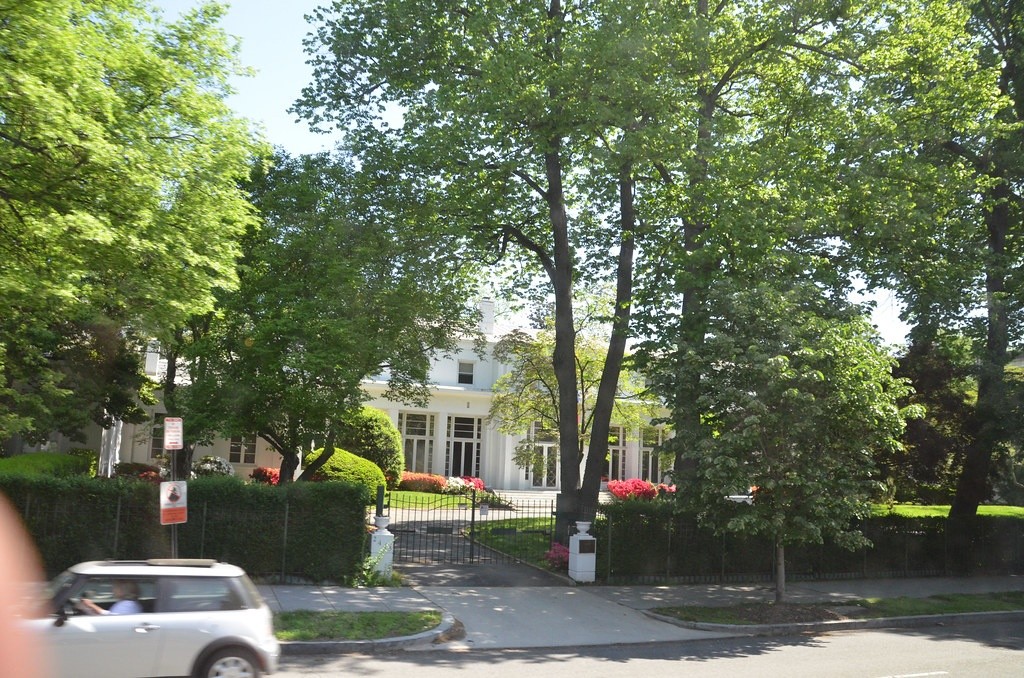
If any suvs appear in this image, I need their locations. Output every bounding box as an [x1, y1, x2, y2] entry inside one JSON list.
[[15, 559, 280, 678]]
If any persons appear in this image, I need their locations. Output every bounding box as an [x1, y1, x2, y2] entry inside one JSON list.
[[81, 583, 142, 616]]
